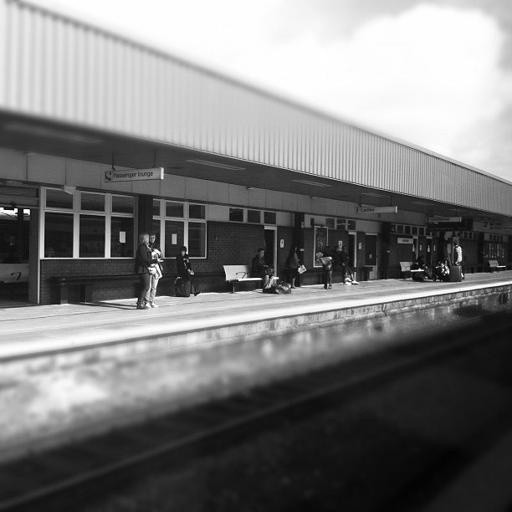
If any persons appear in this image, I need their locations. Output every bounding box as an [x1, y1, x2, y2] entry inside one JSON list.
[[409, 254, 450, 282], [144, 232, 164, 309], [175, 246, 201, 298], [284, 242, 306, 289], [339, 245, 360, 286], [135, 232, 164, 310], [453, 239, 465, 280], [319, 245, 336, 289], [250, 247, 279, 294]]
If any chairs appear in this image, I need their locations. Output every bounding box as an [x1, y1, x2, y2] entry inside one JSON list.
[[137, 303, 158, 308]]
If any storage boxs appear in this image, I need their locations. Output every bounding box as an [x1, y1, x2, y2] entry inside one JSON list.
[[488, 259, 507, 271], [222, 263, 263, 293], [399, 261, 424, 276]]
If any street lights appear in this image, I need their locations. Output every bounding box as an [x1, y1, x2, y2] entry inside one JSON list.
[[275, 281, 291, 294], [297, 265, 306, 274]]
